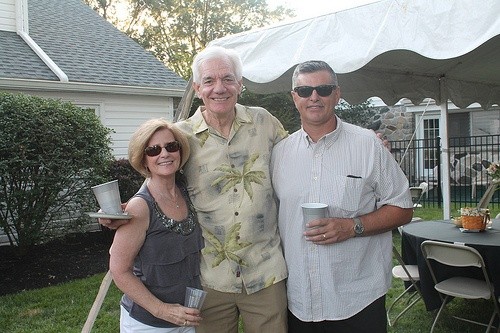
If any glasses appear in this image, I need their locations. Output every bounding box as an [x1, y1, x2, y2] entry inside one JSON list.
[[143, 141, 180, 156], [294, 85, 337, 98]]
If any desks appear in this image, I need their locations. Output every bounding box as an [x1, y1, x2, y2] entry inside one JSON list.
[[401, 220, 500, 311]]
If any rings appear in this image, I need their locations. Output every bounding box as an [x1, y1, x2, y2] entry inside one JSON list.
[[323, 233, 326, 241], [184, 321, 187, 327]]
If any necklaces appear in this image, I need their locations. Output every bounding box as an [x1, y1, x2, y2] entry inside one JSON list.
[[168, 185, 180, 209]]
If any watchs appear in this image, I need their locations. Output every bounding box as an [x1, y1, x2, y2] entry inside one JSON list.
[[352, 217, 365, 237]]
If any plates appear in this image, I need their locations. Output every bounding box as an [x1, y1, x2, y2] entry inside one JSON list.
[[84, 211, 138, 219]]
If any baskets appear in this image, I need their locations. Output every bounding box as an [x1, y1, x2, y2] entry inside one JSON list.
[[461, 216, 488, 231]]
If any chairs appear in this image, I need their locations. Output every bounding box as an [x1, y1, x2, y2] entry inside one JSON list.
[[420, 240, 500, 333], [387, 244, 422, 328], [408, 186, 425, 212]]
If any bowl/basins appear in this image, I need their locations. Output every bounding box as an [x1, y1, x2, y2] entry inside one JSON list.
[[453, 217, 462, 227]]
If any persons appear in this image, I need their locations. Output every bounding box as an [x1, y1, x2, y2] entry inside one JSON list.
[[97, 47, 392, 333], [270, 61, 412, 333], [109, 119, 204, 333]]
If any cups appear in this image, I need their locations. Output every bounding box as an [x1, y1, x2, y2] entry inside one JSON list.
[[184, 287, 208, 311], [91, 180, 123, 214], [300, 203, 328, 238], [487, 219, 493, 229]]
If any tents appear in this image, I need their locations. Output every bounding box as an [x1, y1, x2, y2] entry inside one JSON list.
[[175, 0, 500, 220]]
